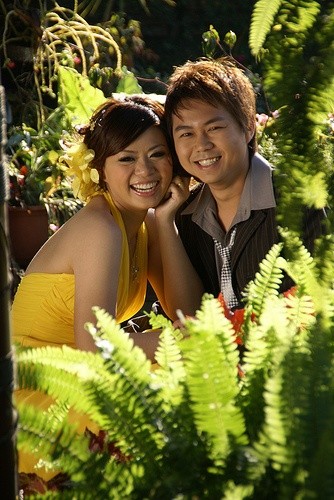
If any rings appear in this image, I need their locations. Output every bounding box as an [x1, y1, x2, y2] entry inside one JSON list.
[[177, 182, 182, 188]]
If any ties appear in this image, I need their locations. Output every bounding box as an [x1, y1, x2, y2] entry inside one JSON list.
[[212, 228, 239, 311]]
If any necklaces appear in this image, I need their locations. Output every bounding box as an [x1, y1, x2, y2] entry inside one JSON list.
[[129, 245, 143, 275]]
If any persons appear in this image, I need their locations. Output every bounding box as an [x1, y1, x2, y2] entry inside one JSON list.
[[9, 96, 207, 363], [168, 59, 320, 316]]
